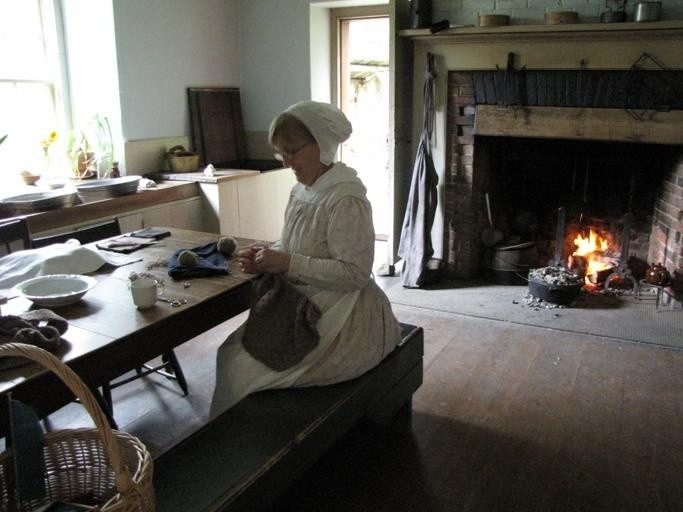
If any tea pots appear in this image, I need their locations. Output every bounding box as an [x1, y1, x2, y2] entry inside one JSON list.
[[645, 261, 671, 285]]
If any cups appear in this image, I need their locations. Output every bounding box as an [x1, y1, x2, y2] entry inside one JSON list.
[[131, 277, 161, 310]]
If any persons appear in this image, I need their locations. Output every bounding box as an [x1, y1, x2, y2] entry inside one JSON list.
[[204, 100, 399, 422]]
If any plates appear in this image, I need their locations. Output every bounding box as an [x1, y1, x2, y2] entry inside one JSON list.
[[10, 273, 98, 307]]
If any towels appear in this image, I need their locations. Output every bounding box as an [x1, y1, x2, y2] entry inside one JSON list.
[[0, 238, 144, 300]]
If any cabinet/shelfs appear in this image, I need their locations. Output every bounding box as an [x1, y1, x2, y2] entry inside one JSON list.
[[115, 196, 205, 234]]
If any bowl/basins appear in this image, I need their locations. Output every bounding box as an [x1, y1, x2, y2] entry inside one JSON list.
[[75, 173, 143, 201], [1, 189, 78, 214]]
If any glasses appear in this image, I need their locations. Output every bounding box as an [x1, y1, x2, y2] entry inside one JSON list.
[[271, 141, 312, 163]]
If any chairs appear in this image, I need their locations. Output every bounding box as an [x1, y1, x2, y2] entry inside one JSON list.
[[22, 217, 189, 429]]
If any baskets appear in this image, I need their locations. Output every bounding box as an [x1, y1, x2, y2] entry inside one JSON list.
[[165, 145, 201, 173], [1, 340, 158, 511]]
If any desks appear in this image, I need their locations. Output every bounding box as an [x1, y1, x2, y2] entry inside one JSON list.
[[0, 226, 275, 437]]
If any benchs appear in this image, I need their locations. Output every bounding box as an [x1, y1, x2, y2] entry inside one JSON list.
[[153, 322, 424, 512]]
